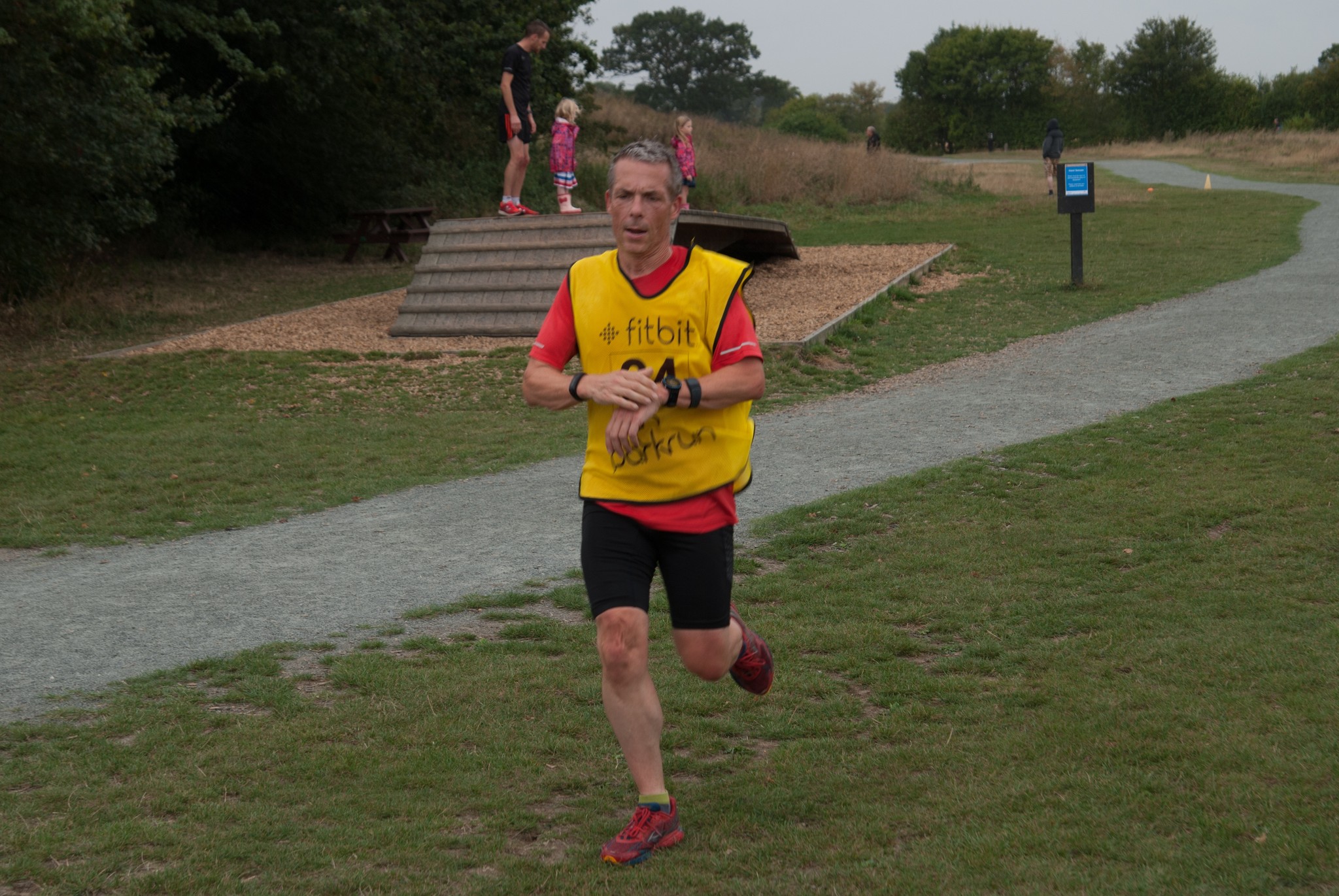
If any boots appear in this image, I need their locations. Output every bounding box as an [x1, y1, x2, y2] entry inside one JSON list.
[[557, 193, 581, 213]]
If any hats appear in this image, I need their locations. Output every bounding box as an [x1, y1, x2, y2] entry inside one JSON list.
[[1047, 119, 1059, 129]]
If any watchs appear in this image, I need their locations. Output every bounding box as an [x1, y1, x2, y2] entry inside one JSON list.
[[569, 373, 586, 402], [661, 377, 681, 407]]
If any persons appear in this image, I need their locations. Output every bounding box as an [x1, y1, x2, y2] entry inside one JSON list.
[[522, 142, 772, 866], [669, 116, 697, 210], [550, 98, 581, 213], [498, 20, 553, 216], [1042, 120, 1064, 195], [866, 126, 881, 154], [1274, 118, 1280, 131]]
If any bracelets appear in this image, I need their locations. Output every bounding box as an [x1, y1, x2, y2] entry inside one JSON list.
[[684, 378, 702, 408], [527, 111, 532, 114]]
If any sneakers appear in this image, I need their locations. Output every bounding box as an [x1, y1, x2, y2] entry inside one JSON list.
[[600, 796, 683, 867], [498, 201, 522, 216], [726, 602, 773, 696], [516, 203, 539, 215]]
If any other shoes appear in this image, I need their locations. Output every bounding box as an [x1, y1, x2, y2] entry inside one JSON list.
[[680, 204, 689, 209]]
